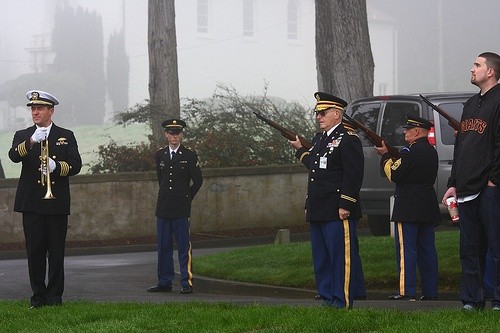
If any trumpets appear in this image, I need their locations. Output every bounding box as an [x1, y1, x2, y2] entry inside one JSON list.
[[38, 136, 58, 199]]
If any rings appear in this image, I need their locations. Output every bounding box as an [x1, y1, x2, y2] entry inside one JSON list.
[[345, 215, 348, 218]]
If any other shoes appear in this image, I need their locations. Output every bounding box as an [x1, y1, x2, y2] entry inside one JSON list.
[[388, 293, 416, 300], [420, 296, 440, 300], [463, 305, 485, 316], [491, 302, 500, 311]]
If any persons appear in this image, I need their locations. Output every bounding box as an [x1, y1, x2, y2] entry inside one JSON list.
[[441, 52, 500, 312], [8, 90, 83, 309], [145, 118, 203, 295], [287, 118, 359, 169], [304, 92, 364, 310], [374, 113, 441, 301]]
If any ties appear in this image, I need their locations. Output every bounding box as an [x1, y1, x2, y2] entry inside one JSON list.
[[171, 151, 175, 159], [321, 131, 327, 140]]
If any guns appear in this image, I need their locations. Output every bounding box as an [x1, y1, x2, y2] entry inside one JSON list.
[[418, 93, 461, 132], [252, 109, 311, 150], [343, 112, 397, 158]]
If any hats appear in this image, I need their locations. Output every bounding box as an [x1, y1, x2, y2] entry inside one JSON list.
[[26, 90, 59, 107], [161, 119, 186, 134], [402, 115, 434, 130], [314, 92, 347, 112]]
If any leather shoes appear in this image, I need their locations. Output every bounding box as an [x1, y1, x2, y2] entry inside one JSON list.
[[180, 285, 192, 293], [147, 284, 172, 292]]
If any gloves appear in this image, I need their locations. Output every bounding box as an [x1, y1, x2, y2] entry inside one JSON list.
[[31, 127, 48, 143], [41, 157, 56, 173]]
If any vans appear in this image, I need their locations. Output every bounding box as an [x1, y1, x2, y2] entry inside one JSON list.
[[344, 92, 481, 237]]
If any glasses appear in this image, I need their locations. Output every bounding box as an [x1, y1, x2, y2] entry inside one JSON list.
[[316, 111, 327, 116]]
